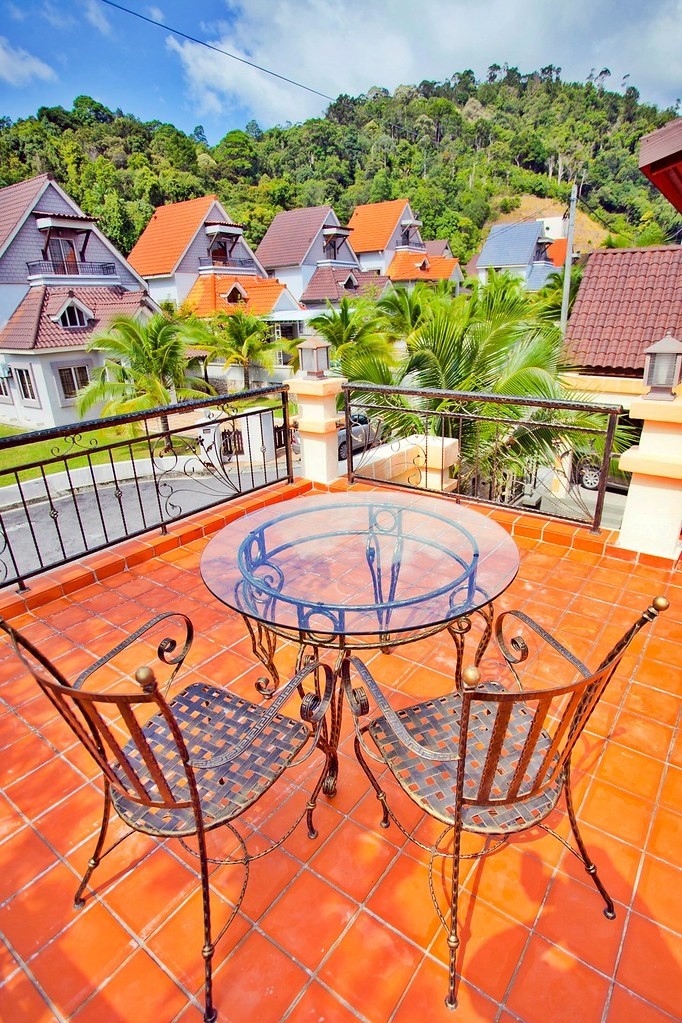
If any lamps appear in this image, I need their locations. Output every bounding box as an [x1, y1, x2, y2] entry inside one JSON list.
[[642, 331, 682, 401], [296, 335, 332, 379]]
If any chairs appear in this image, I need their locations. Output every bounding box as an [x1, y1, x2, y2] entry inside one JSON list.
[[0, 611, 339, 1023], [342, 598, 671, 1010]]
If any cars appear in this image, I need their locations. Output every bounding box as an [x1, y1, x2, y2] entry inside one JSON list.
[[292, 414, 392, 461], [570, 443, 632, 494]]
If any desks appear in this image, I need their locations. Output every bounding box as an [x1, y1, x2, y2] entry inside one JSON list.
[[200, 492, 520, 796]]
